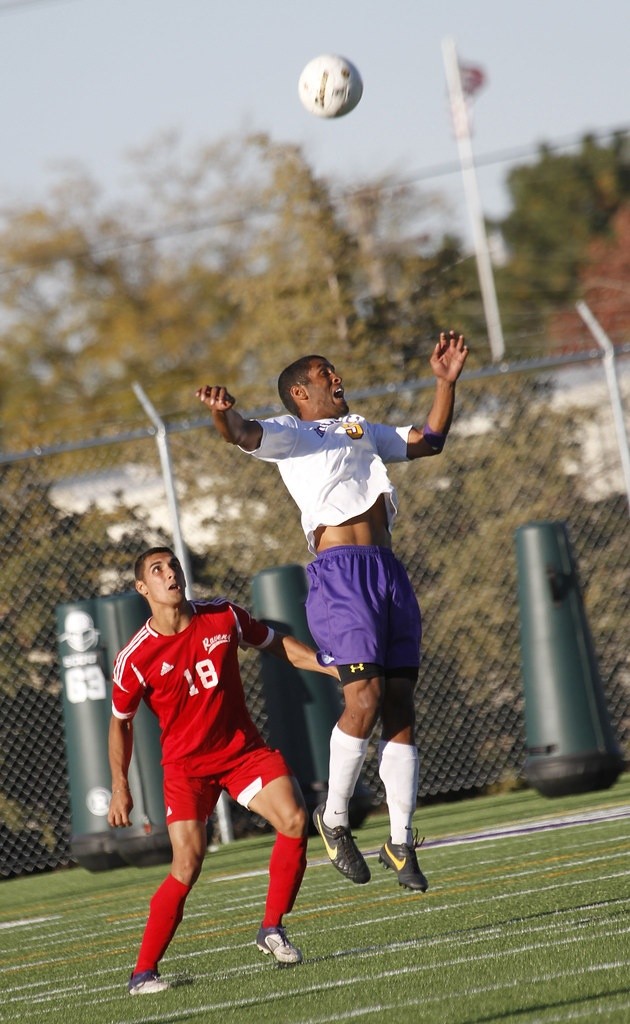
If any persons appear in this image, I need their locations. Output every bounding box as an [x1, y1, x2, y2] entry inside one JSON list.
[[196, 326, 472, 893], [106, 546, 340, 998]]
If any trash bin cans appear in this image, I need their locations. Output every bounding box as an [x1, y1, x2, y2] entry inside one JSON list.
[[247, 562, 368, 832], [58, 590, 173, 871], [517, 520, 625, 799]]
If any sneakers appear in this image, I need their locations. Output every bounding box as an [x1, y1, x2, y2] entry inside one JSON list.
[[312, 801, 371, 884], [128, 971, 172, 996], [378, 826, 429, 894], [254, 922, 303, 964]]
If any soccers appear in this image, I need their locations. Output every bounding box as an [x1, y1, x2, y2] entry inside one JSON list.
[[298, 53, 364, 120]]
